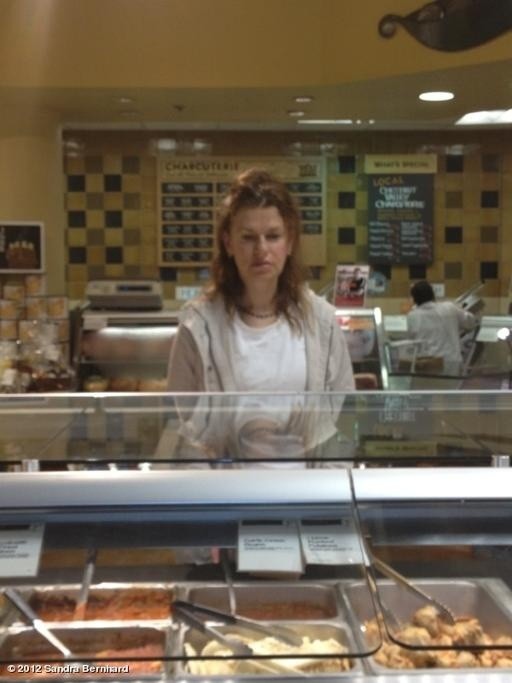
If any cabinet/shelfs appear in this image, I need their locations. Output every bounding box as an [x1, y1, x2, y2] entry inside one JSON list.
[[0, 308, 512, 683]]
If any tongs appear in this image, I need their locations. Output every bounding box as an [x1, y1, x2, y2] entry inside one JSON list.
[[368, 552, 457, 632], [169, 598, 308, 676]]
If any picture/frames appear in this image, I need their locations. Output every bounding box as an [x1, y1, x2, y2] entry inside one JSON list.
[[0, 221, 46, 275]]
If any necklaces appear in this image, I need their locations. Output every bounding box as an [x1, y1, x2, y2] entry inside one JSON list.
[[243, 426, 276, 436], [236, 304, 279, 318]]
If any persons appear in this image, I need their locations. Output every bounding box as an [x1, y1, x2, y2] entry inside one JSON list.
[[345, 267, 365, 299], [164, 164, 361, 393], [403, 280, 481, 378], [172, 395, 347, 461]]
